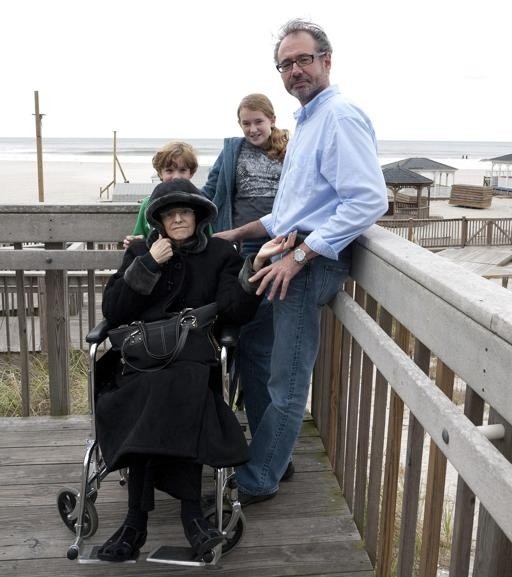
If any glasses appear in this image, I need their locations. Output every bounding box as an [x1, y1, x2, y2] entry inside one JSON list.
[[276, 52, 325, 73]]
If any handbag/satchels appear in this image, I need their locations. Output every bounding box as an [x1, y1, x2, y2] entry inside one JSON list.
[[107, 301, 221, 377]]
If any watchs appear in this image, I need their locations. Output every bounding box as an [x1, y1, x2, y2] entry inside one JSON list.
[[294, 247, 310, 266]]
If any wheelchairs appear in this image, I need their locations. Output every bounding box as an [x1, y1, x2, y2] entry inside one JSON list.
[[56, 319, 247, 568]]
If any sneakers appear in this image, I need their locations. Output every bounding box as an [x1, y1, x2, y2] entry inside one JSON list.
[[202, 461, 294, 509], [184, 517, 224, 554], [96, 525, 147, 562]]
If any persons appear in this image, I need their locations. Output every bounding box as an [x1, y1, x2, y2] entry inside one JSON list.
[[211, 18, 388, 506], [96, 180, 297, 565], [201, 93, 290, 258], [123, 140, 212, 250]]
